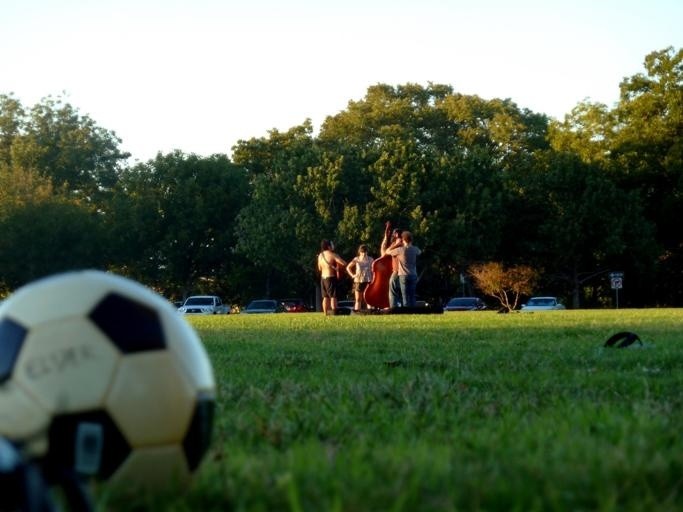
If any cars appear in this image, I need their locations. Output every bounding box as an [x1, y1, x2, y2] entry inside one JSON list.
[[444, 297, 487, 311], [241, 299, 431, 314], [520, 296, 565, 310]]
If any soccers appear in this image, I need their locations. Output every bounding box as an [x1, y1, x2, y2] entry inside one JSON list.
[[0, 271, 215, 511]]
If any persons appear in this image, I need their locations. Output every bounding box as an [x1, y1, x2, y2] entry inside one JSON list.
[[385, 230, 422, 308], [379, 228, 402, 314], [345, 244, 374, 313], [316, 237, 348, 318]]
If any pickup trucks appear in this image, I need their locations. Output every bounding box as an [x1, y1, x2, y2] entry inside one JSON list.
[[177, 295, 230, 315]]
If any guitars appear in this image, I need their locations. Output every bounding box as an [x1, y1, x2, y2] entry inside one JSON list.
[[334, 262, 343, 279]]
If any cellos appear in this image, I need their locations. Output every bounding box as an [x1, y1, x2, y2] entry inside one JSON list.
[[364, 221, 392, 308]]
